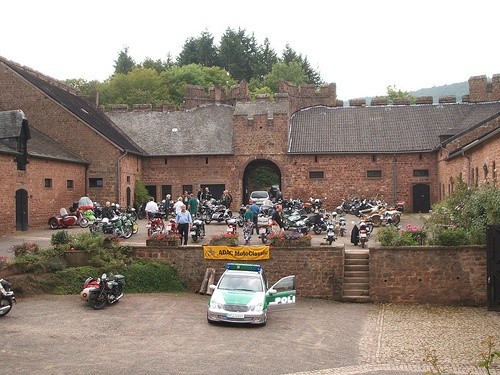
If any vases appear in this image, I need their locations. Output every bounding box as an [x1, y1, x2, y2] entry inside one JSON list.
[[145, 238, 181, 247], [210, 238, 238, 248]]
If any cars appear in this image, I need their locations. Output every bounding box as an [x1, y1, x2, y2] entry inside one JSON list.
[[207, 263, 296, 326]]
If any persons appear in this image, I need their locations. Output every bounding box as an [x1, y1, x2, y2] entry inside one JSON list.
[[164, 187, 283, 236], [176, 204, 193, 244], [145, 197, 159, 224]]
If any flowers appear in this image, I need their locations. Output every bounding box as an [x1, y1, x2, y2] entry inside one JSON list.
[[147, 230, 181, 240], [266, 230, 312, 247], [376, 225, 430, 246], [212, 234, 237, 239]]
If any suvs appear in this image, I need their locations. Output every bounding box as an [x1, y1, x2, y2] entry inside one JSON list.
[[248, 191, 270, 205]]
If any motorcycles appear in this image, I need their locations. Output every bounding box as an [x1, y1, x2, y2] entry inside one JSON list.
[[71, 200, 138, 239], [227, 211, 244, 227], [50, 207, 89, 229], [138, 193, 232, 225], [191, 214, 206, 243], [0, 280, 16, 316], [260, 197, 406, 249], [81, 273, 126, 311], [145, 212, 166, 234], [227, 219, 239, 237]]
